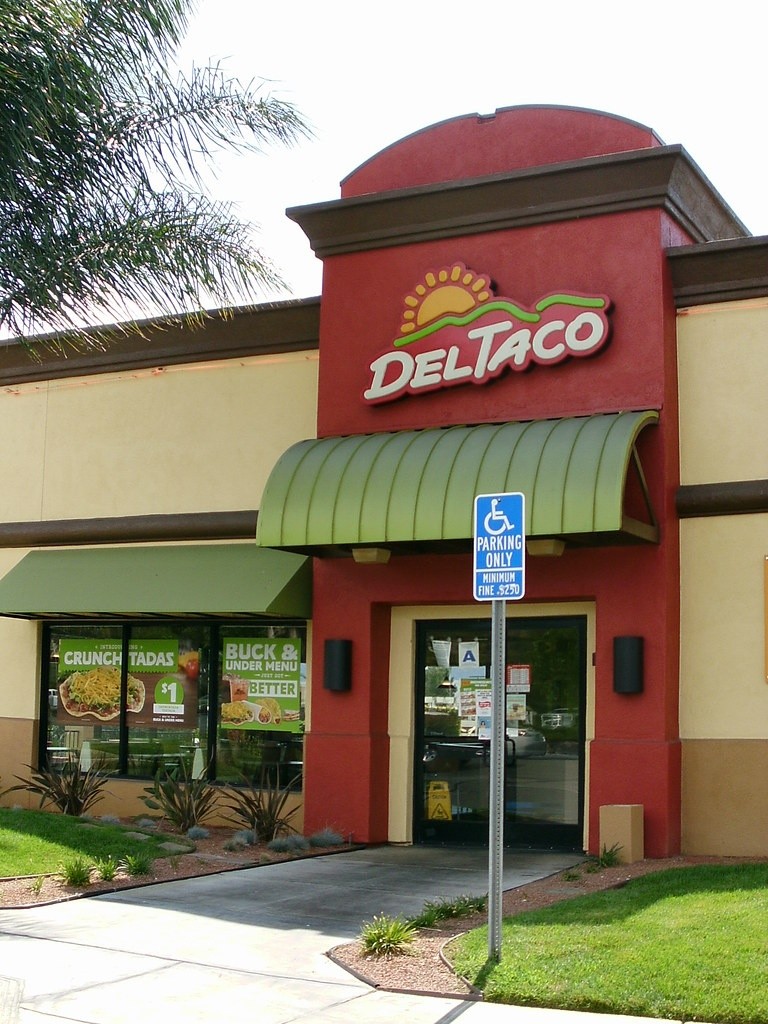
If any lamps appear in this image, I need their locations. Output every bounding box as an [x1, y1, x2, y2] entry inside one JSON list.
[[612, 635, 644, 694], [352, 548, 391, 565], [323, 639, 352, 691], [526, 539, 565, 557]]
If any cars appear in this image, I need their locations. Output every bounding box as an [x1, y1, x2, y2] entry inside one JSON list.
[[504, 718, 549, 759]]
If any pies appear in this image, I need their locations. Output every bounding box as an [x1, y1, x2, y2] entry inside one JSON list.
[[59, 671, 145, 721], [220, 700, 281, 725]]
